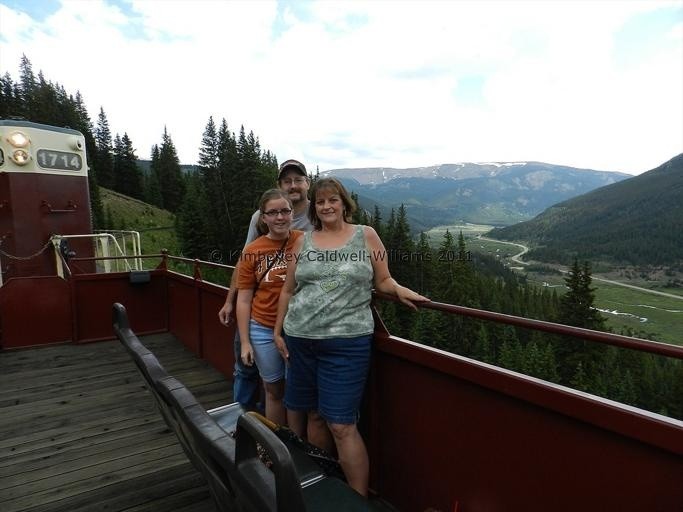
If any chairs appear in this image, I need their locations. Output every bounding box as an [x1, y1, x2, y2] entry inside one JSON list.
[[108, 301, 324, 498], [233, 415, 383, 512]]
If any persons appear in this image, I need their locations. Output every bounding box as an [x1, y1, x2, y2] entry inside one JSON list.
[[273, 176, 431, 498], [236, 188, 306, 428], [218, 159, 310, 414]]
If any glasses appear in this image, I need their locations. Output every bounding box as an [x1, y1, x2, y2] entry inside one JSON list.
[[263, 208, 292, 216], [281, 177, 307, 184]]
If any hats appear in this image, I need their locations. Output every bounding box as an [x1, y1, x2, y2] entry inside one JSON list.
[[278, 159, 307, 180]]
